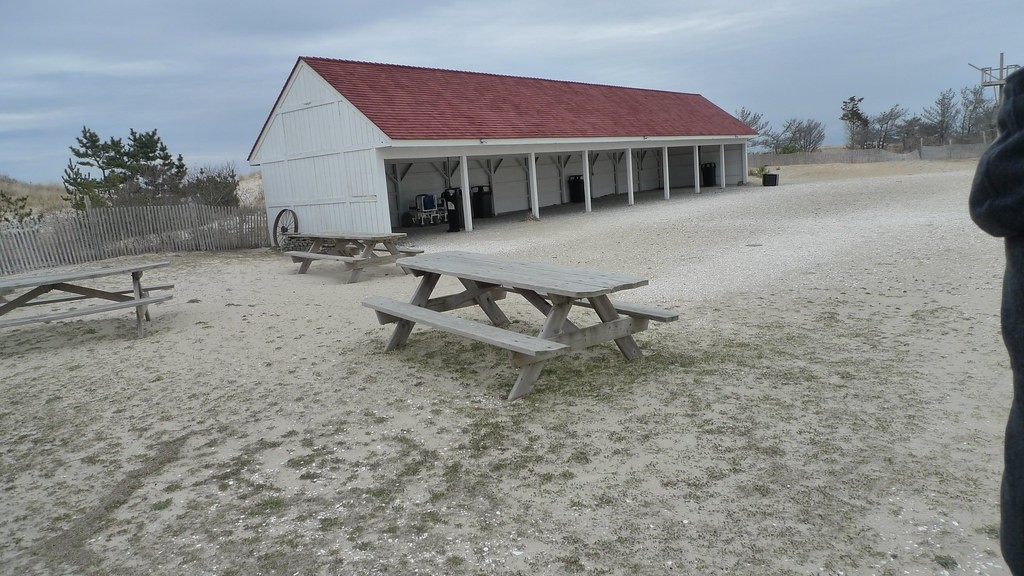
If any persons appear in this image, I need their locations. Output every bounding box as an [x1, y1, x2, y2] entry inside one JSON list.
[[969, 67, 1024, 576]]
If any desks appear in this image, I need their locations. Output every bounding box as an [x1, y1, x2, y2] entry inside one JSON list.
[[287, 231, 413, 283], [383, 250, 652, 397], [0, 260, 170, 339]]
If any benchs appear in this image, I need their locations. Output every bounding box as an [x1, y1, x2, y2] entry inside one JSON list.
[[0, 292, 173, 329], [286, 251, 367, 260], [362, 296, 572, 355], [499, 285, 681, 322], [322, 246, 424, 254], [0, 284, 178, 298]]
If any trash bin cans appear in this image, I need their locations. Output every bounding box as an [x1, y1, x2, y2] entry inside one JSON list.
[[701, 162, 716, 186], [471, 185, 492, 217], [567, 175, 585, 202]]
